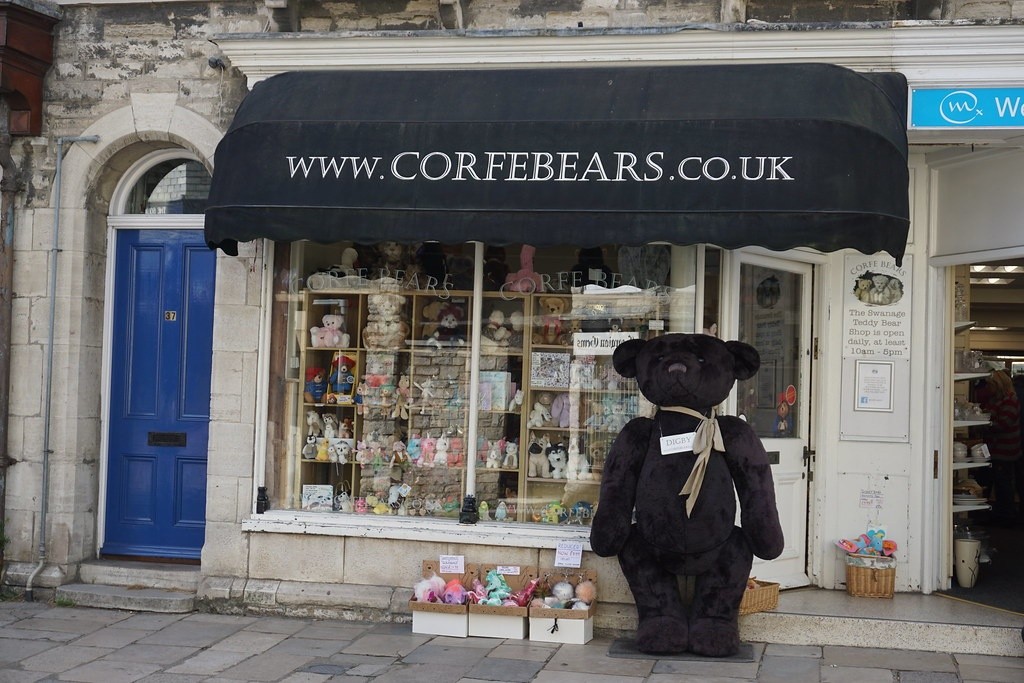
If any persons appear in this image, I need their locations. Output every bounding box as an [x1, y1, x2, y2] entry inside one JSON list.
[[972, 368, 1024, 529]]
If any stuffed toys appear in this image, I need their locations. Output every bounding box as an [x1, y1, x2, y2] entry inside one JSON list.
[[305, 241, 657, 526], [414, 570, 596, 611], [772, 398, 793, 438], [588, 334, 785, 661]]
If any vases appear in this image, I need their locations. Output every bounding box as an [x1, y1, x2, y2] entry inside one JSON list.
[[955, 539, 981, 589]]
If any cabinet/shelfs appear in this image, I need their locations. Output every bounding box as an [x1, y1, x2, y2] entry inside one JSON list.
[[293, 288, 670, 529], [953, 321, 996, 580]]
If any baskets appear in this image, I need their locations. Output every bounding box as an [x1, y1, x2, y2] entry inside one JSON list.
[[737, 577, 780, 615], [845, 552, 895, 599]]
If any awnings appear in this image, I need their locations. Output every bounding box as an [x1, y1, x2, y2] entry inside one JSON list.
[[202, 67, 910, 267]]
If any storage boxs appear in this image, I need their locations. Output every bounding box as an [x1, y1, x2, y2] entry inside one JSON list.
[[408, 560, 598, 645]]
